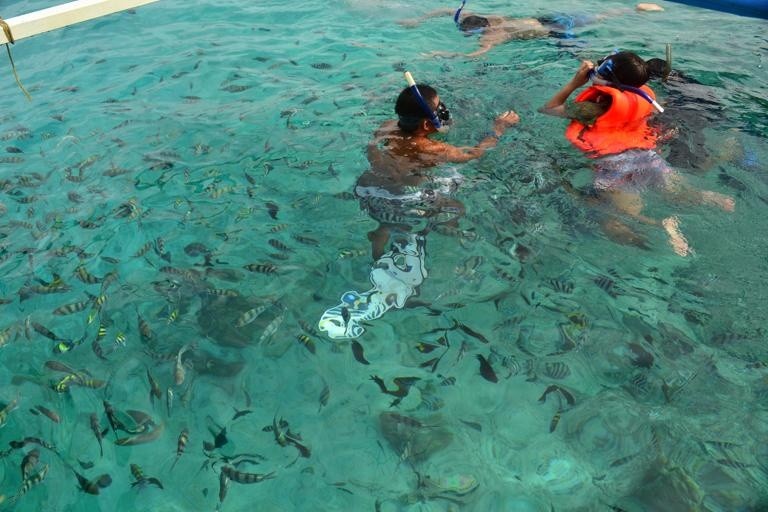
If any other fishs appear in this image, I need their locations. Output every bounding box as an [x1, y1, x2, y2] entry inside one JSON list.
[[1, 25, 768, 512]]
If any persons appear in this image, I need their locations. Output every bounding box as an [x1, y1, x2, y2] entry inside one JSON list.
[[358, 84, 523, 260], [541, 51, 735, 256], [392, 2, 664, 58], [644, 58, 757, 192]]
